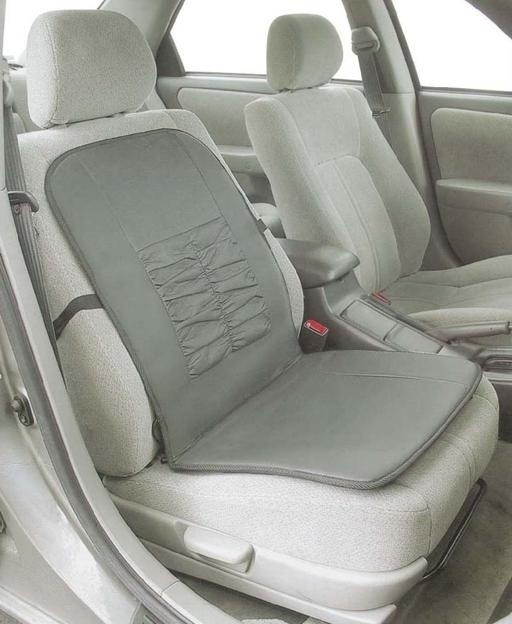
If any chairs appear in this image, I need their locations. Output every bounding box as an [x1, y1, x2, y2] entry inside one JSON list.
[[17, 9, 500, 619], [5, 69, 282, 237], [244, 14, 511, 420]]
